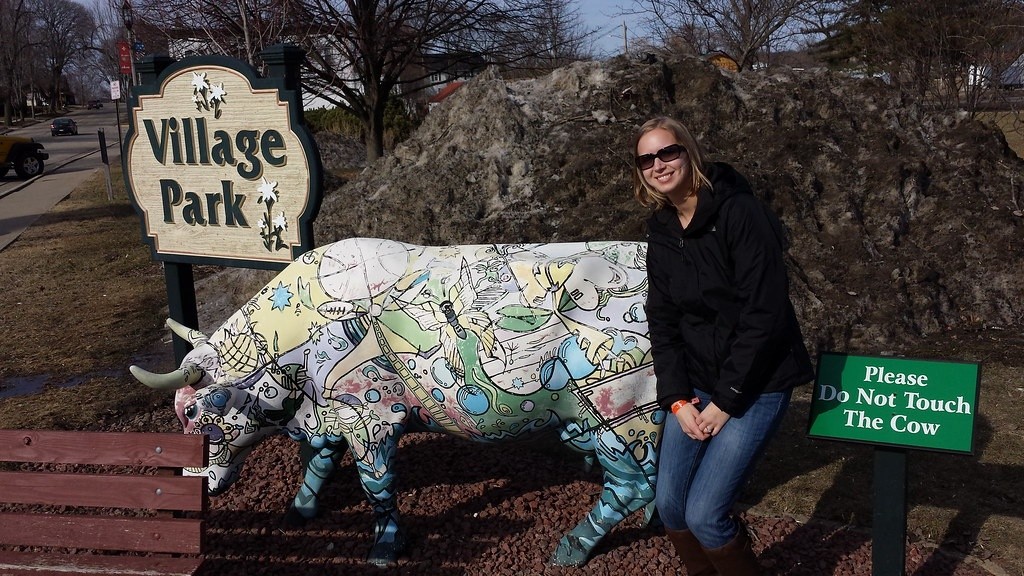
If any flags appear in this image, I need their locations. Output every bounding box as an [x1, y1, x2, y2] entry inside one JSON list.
[[117, 42, 132, 73]]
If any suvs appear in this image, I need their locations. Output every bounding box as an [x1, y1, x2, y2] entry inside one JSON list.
[[0, 134, 49, 179]]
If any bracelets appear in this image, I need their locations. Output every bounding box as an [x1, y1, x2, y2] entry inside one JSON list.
[[672, 399, 689, 414]]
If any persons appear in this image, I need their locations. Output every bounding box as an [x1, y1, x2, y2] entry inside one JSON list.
[[630, 117, 817, 576]]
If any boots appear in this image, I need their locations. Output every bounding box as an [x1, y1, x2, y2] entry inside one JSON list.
[[703, 520, 762, 576], [662, 525, 717, 576]]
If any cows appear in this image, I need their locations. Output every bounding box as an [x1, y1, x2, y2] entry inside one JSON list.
[[127, 237, 666, 570]]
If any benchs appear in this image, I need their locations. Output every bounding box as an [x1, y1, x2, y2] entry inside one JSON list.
[[0, 427, 210, 575]]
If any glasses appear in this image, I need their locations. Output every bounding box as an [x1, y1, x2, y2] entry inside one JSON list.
[[635, 143, 686, 171]]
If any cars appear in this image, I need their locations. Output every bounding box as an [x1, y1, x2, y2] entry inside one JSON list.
[[86, 99, 103, 110], [51, 118, 78, 136]]
